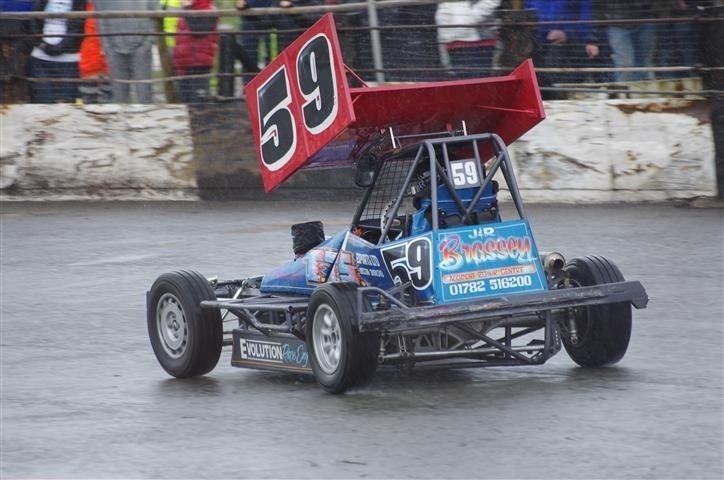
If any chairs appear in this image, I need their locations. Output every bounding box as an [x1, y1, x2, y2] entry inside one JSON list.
[[414, 180, 502, 230]]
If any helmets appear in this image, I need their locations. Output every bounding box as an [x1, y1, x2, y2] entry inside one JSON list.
[[417, 170, 442, 189]]
